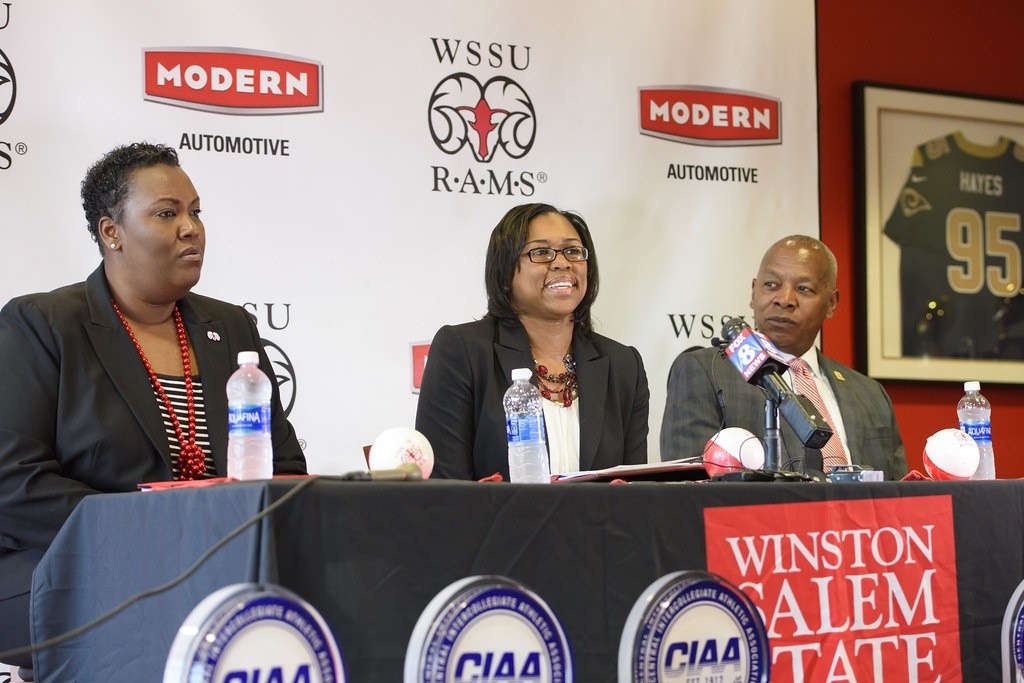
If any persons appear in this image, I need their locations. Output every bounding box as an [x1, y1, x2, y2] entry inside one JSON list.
[[413, 204, 649, 483], [659, 234, 909, 479], [0, 143, 306, 668]]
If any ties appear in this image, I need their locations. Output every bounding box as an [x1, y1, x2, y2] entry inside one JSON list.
[[786, 357, 847, 474]]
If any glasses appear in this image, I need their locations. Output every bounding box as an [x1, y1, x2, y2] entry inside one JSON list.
[[521, 246, 590, 263]]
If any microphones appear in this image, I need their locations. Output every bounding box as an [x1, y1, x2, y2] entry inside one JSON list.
[[722, 317, 833, 448], [343, 462, 423, 481]]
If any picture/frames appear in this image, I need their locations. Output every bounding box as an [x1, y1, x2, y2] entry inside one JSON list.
[[850, 79, 1024, 387]]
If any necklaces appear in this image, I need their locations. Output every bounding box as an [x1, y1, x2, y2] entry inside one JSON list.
[[532, 348, 579, 407], [112, 300, 205, 480]]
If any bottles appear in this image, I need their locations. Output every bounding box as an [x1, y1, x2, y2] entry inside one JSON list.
[[956, 381, 997, 480], [226, 351, 274, 481], [503, 367, 551, 484]]
